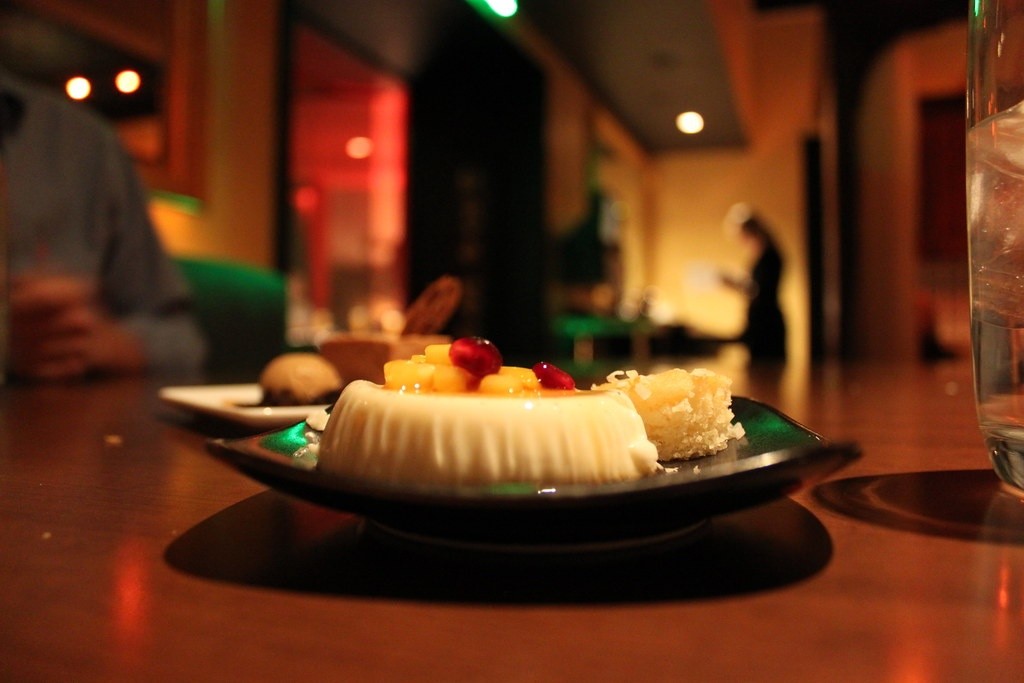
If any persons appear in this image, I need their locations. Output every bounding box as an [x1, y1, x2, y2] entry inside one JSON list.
[[0, 72, 205, 384], [712, 205, 788, 370]]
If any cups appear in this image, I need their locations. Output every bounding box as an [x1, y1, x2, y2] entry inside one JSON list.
[[967, 1, 1024, 501]]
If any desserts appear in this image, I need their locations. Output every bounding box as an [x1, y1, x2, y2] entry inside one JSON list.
[[318, 335, 660, 484]]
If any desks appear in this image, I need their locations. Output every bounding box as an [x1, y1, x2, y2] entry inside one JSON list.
[[0, 348, 1024, 683]]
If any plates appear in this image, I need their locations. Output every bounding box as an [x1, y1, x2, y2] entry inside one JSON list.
[[160, 382, 329, 424], [205, 394, 859, 541]]
